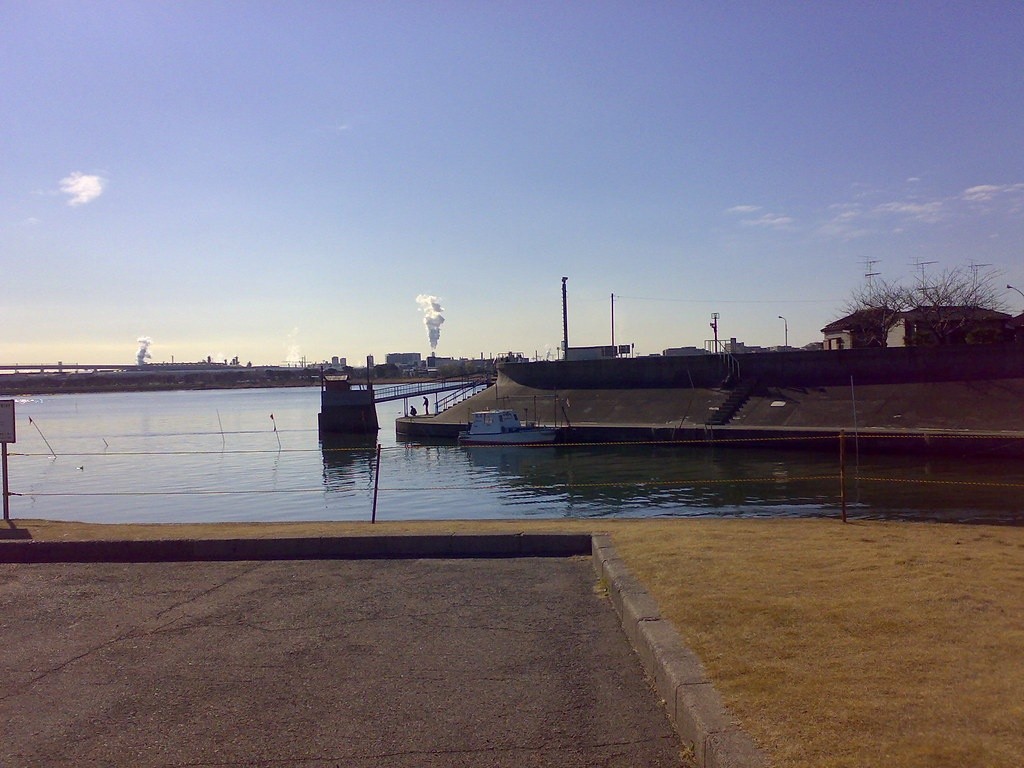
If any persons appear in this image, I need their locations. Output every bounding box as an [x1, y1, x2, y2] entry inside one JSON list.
[[423, 396, 429, 415], [409, 406, 417, 416]]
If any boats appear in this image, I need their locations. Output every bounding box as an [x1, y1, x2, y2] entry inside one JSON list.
[[318, 357, 379, 434], [457, 406, 557, 445]]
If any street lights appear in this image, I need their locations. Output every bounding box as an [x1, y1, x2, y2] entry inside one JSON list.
[[779, 316, 788, 347]]
